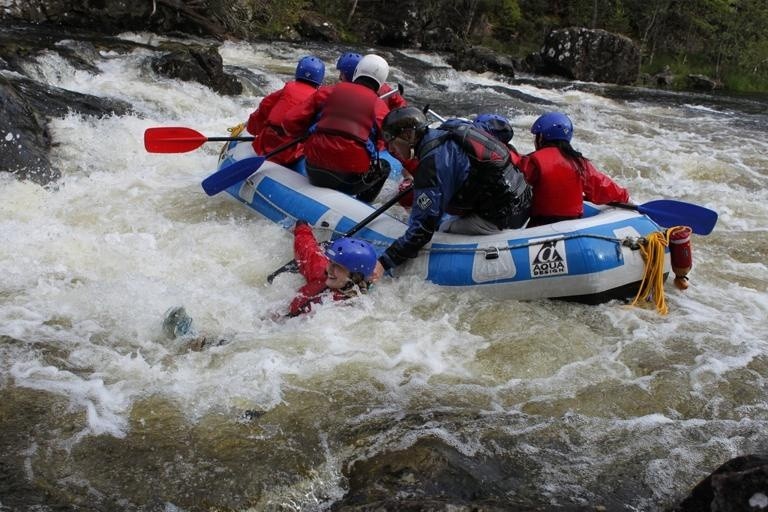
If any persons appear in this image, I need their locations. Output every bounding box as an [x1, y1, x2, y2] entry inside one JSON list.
[[336, 50, 407, 112], [526, 111, 632, 228], [282, 53, 391, 204], [247, 54, 325, 166], [163, 221, 377, 357], [398, 113, 520, 209], [366, 105, 532, 285]]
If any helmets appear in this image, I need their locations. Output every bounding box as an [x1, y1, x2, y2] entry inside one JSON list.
[[324, 236, 376, 281], [335, 50, 364, 76], [350, 53, 390, 94], [294, 54, 325, 87], [529, 109, 573, 142], [379, 105, 428, 144], [472, 110, 514, 144]]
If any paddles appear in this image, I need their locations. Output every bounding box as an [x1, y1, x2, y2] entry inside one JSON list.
[[144, 127, 255, 153], [267, 188, 413, 285], [202, 83, 403, 196], [584, 196, 718, 235]]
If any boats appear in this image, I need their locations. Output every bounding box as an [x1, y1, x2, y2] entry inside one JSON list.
[[217, 117, 676, 307]]
